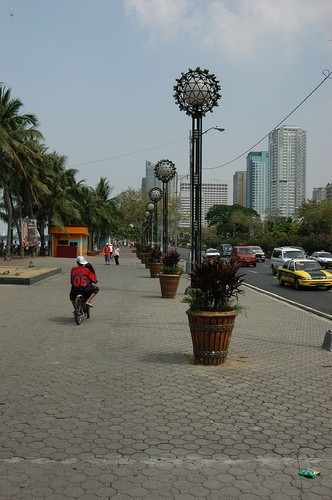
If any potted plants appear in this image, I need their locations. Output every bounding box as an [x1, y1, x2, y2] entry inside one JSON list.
[[136, 242, 246, 365]]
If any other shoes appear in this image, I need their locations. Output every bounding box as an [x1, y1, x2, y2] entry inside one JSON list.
[[85, 302, 93, 307]]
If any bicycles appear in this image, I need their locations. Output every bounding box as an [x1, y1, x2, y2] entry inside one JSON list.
[[72, 280, 98, 325]]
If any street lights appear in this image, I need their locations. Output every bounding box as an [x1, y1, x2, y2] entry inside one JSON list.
[[190, 127, 226, 272], [174, 66, 223, 273], [155, 160, 178, 254], [145, 186, 162, 248]]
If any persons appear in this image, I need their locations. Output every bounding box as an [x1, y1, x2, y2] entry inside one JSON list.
[[108, 244, 114, 257], [14, 241, 18, 248], [114, 245, 120, 265], [0, 239, 4, 252], [131, 241, 133, 246], [69, 256, 99, 314], [104, 243, 111, 265]]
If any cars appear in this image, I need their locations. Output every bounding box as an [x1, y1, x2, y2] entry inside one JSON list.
[[310, 252, 332, 269], [204, 249, 222, 259], [219, 244, 232, 257], [276, 258, 332, 289]]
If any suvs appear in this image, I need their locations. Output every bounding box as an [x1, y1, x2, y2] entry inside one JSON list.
[[271, 247, 308, 274], [231, 245, 257, 268], [249, 246, 265, 262]]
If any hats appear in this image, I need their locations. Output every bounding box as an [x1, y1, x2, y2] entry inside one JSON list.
[[77, 256, 88, 265]]
